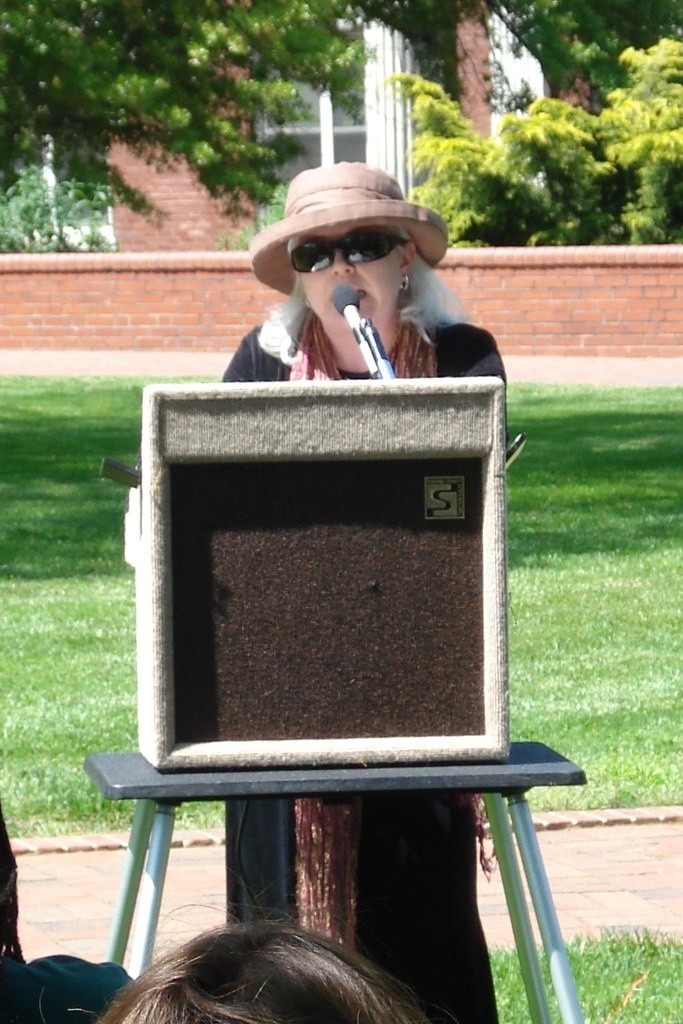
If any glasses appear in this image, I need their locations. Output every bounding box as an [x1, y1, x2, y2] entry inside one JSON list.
[[289, 228, 413, 273]]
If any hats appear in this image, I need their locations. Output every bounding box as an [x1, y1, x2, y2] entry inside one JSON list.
[[247, 162, 449, 298]]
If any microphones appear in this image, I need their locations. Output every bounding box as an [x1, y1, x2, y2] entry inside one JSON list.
[[332, 283, 382, 379]]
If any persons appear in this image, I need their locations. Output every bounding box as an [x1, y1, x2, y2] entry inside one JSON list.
[[98, 920, 459, 1024], [221, 161, 508, 1024]]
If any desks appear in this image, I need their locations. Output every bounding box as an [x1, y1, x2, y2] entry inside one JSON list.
[[83, 742, 587, 1024]]
